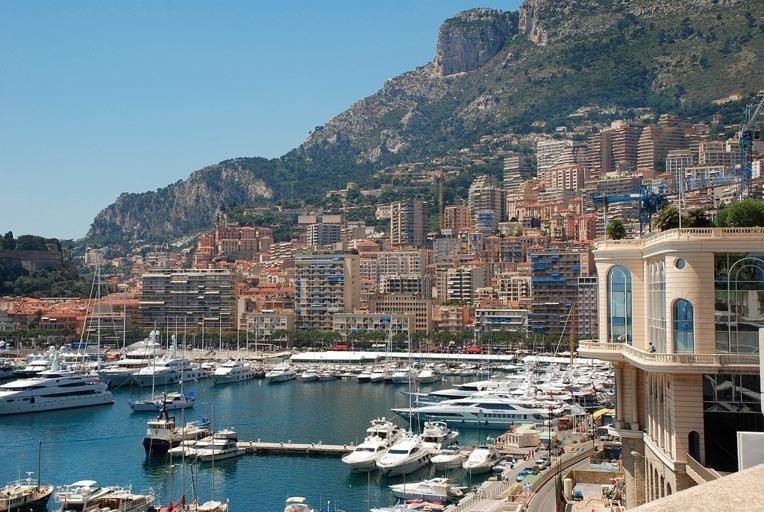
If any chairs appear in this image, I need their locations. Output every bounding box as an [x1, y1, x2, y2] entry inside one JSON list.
[[601, 475, 626, 512]]
